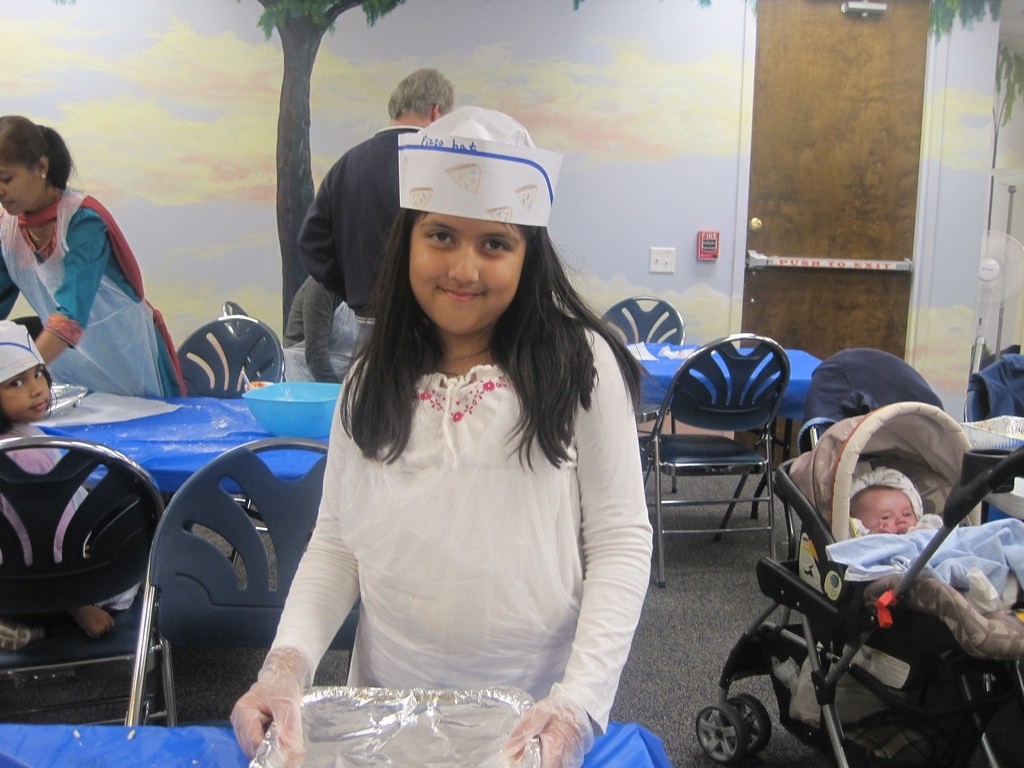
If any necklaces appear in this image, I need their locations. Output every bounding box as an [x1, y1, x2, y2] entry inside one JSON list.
[[27, 218, 49, 243]]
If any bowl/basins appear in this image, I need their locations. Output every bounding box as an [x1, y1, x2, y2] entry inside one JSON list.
[[241, 380, 342, 439]]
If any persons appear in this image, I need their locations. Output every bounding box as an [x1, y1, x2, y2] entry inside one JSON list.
[[853, 467, 1018, 618], [284, 67, 453, 384], [228, 110, 655, 768], [0, 322, 141, 651], [0, 116, 189, 400]]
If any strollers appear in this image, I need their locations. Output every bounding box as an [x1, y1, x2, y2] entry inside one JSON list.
[[694, 444, 1024, 768]]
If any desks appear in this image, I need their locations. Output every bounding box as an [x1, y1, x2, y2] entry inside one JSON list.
[[615, 340, 823, 562], [0, 716, 674, 768], [32, 392, 333, 569]]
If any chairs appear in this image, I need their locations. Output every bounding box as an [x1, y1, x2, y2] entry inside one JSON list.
[[0, 437, 178, 731], [121, 436, 365, 731], [176, 298, 287, 397], [598, 296, 688, 493], [637, 333, 789, 590], [965, 355, 1024, 526]]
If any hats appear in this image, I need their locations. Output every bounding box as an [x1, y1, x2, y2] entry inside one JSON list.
[[0, 320, 46, 384], [398, 106, 564, 227]]
